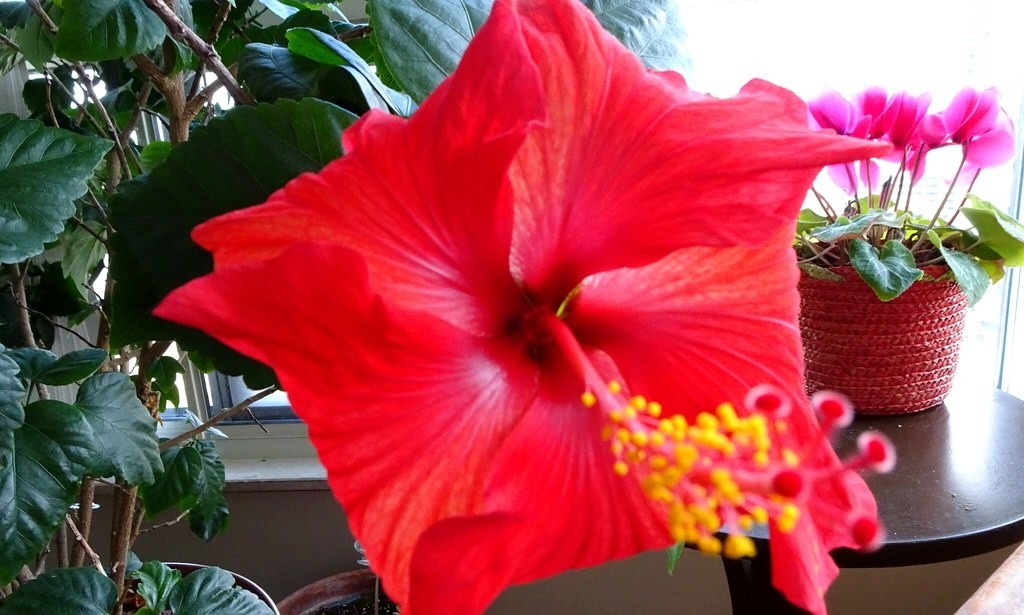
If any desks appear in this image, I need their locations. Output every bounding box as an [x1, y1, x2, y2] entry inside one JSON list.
[[685, 384, 1024, 615]]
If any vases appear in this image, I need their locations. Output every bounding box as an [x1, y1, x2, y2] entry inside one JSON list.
[[797, 264, 968, 417]]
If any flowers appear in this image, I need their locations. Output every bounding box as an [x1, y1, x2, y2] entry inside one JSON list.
[[793, 87, 1024, 309], [151, 0, 894, 615]]
